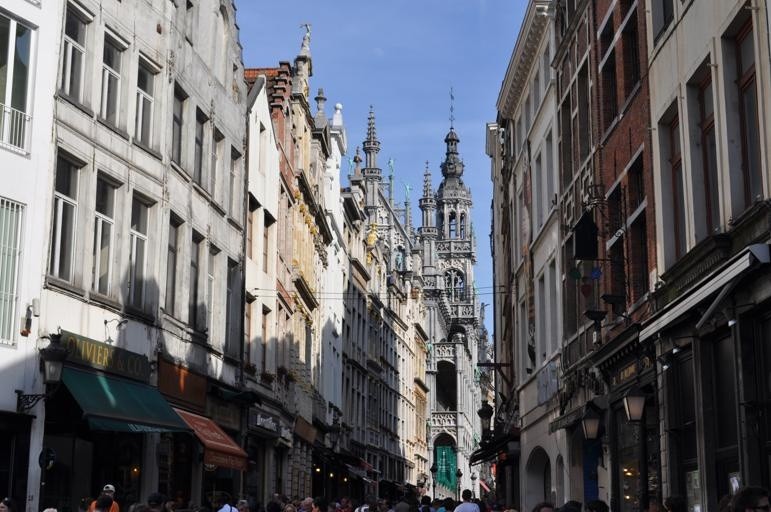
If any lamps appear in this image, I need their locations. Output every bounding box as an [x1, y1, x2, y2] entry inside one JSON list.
[[579, 376, 643, 442], [103, 317, 128, 330], [31, 334, 64, 384]]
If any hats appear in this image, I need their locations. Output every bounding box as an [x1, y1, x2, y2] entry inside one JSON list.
[[103, 484, 115, 492]]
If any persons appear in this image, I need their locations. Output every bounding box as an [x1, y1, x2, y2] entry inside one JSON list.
[[1, 487, 689, 512]]
